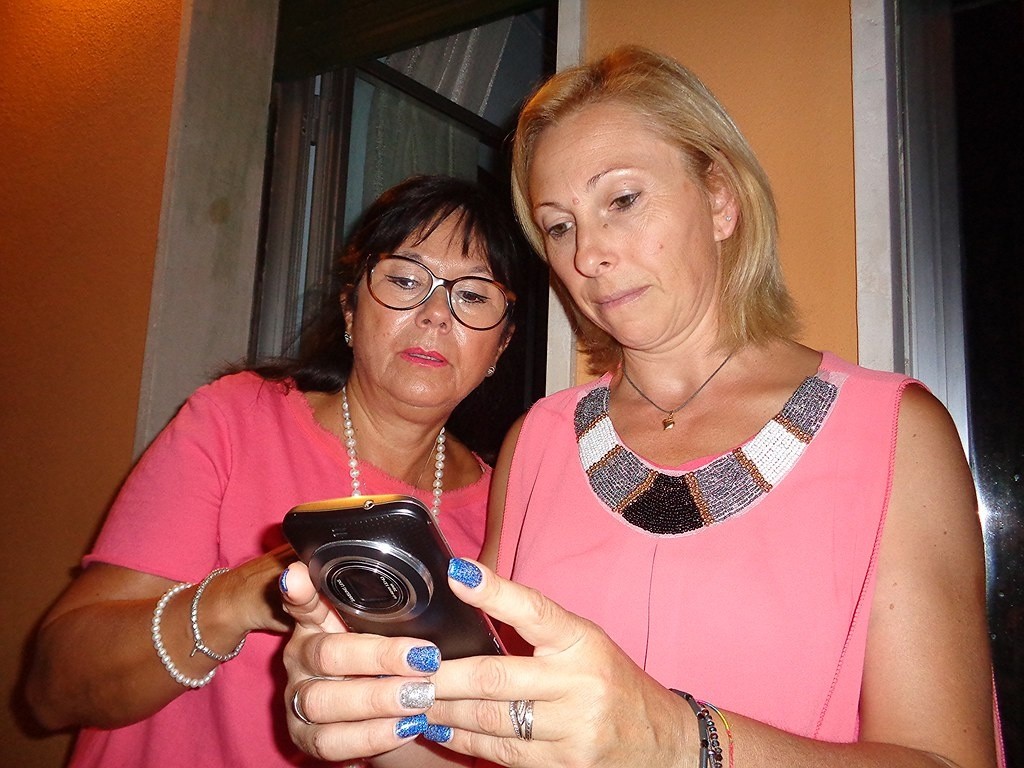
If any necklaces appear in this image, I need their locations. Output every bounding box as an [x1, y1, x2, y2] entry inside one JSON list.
[[342, 388, 445, 526], [362, 439, 440, 498], [624, 348, 736, 431]]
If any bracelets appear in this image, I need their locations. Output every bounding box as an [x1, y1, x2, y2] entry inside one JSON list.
[[700, 704, 724, 768], [152, 581, 215, 688], [668, 686, 708, 768], [697, 700, 733, 768], [190, 568, 244, 661]]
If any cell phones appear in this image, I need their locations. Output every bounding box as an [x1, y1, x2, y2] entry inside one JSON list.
[[283, 492, 511, 660]]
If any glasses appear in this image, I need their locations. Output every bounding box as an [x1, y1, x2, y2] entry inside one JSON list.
[[356, 254, 515, 332]]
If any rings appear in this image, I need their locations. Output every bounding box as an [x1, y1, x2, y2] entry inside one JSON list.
[[292, 676, 323, 724], [510, 700, 534, 741]]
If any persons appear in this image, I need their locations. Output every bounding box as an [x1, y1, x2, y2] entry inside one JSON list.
[[282, 43, 1002, 768], [15, 176, 529, 768]]
